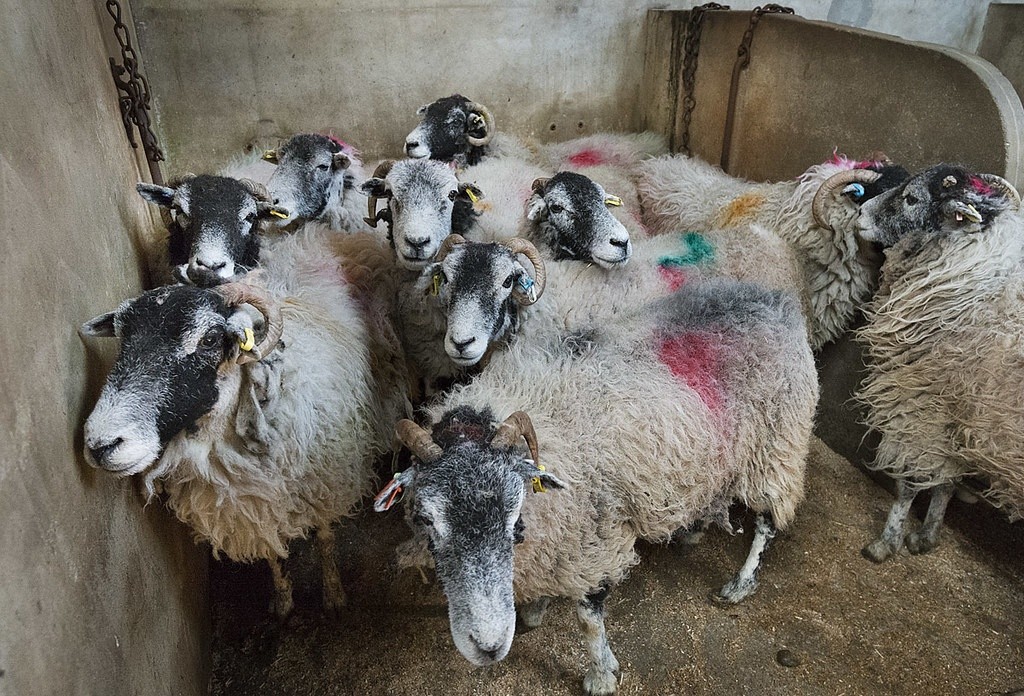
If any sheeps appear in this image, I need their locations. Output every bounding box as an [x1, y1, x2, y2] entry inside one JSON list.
[[374, 273, 819, 695], [82, 89, 1024, 630]]
